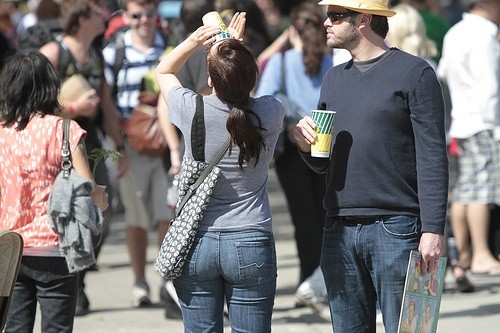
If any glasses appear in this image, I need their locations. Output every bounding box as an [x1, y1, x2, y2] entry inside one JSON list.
[[326, 12, 356, 22], [132, 12, 153, 19]]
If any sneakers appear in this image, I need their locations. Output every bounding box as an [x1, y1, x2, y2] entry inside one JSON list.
[[129, 282, 151, 306]]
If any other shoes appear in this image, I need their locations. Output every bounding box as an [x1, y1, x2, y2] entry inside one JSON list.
[[294, 266, 331, 323], [470, 266, 500, 275], [160, 288, 183, 320], [75, 291, 89, 315], [452, 264, 476, 293]]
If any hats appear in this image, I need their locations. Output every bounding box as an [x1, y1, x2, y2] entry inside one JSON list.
[[10, 11, 38, 39], [318, 0, 397, 17]]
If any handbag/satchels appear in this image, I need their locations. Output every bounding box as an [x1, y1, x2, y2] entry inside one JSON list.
[[151, 152, 223, 281], [49, 116, 109, 275]]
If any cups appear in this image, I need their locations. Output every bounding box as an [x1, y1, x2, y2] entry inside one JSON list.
[[311, 109, 337, 158], [202, 11, 232, 48]]
